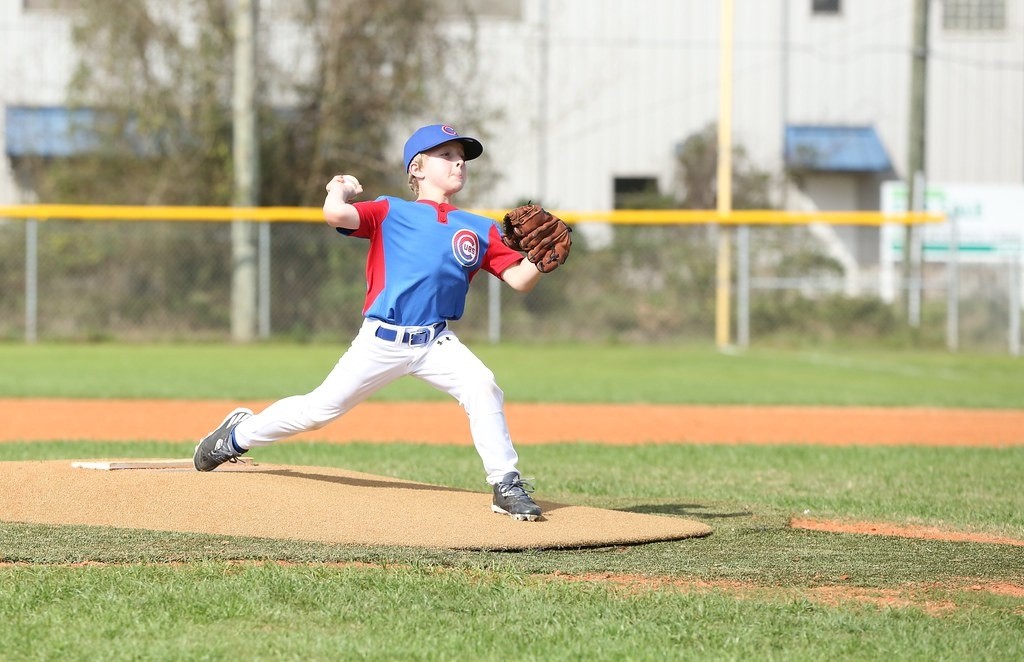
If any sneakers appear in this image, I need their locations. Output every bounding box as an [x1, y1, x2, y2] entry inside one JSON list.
[[193, 408, 254, 472], [491, 472, 542, 521]]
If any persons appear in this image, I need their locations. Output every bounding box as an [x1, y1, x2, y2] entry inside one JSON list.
[[193, 125, 573, 522]]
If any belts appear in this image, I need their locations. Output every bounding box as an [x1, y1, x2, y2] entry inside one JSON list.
[[375, 321, 446, 347]]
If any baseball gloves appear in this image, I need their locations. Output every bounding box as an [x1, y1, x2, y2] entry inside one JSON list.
[[500, 199, 574, 274]]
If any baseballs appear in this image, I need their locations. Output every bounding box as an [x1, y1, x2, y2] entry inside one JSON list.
[[341, 174, 360, 189]]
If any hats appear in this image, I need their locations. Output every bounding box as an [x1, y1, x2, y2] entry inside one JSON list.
[[404, 124, 483, 174]]
